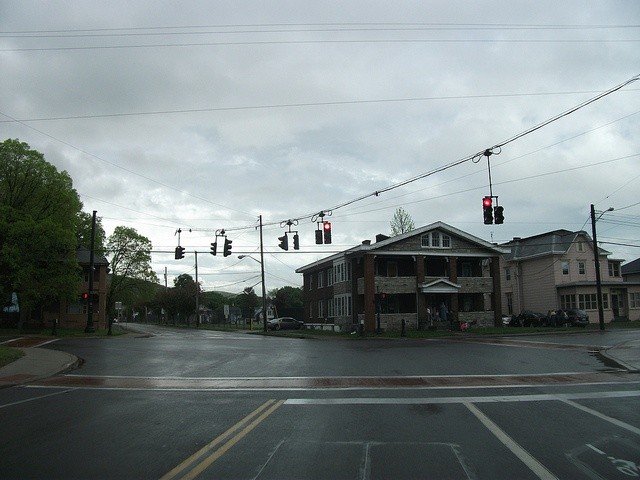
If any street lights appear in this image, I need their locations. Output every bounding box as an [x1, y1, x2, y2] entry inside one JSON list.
[[590, 204, 614, 332], [238, 255, 268, 332]]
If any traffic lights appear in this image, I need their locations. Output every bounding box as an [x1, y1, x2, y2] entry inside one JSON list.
[[315, 230, 323, 244], [224, 239, 232, 257], [293, 235, 299, 249], [494, 206, 504, 224], [175, 246, 185, 259], [324, 223, 331, 244], [210, 242, 216, 255], [84, 291, 90, 304], [278, 235, 288, 250], [483, 198, 493, 224]]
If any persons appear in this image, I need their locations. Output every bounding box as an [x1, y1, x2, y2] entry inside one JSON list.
[[507, 313, 529, 328], [547, 310, 568, 328], [426, 305, 432, 325], [447, 319, 454, 337], [448, 310, 457, 325]]
